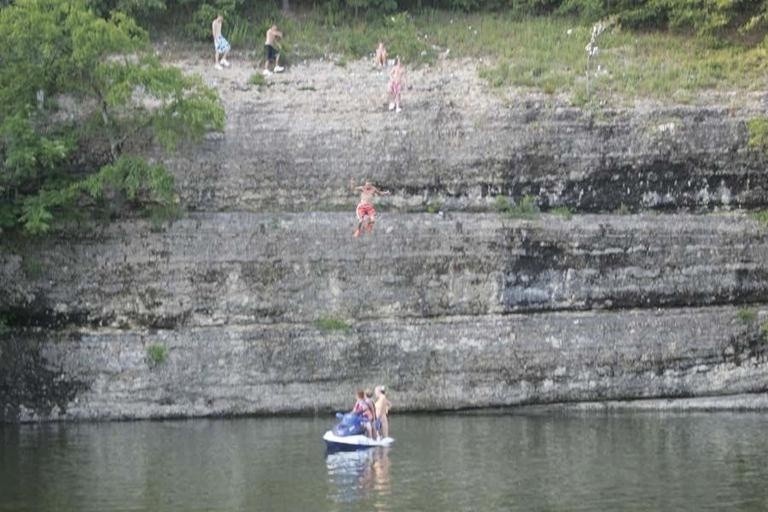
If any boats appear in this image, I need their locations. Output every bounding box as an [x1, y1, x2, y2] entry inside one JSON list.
[[321, 412, 395, 451]]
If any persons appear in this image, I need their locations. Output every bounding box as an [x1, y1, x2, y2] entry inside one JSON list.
[[262, 25, 285, 75], [360, 445, 391, 486], [374, 384, 392, 437], [351, 178, 392, 239], [385, 56, 411, 113], [212, 14, 231, 71], [362, 388, 381, 442], [351, 388, 377, 441], [371, 42, 388, 69]]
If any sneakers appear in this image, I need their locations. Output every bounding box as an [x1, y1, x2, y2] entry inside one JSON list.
[[388, 102, 404, 115], [213, 58, 283, 77]]
